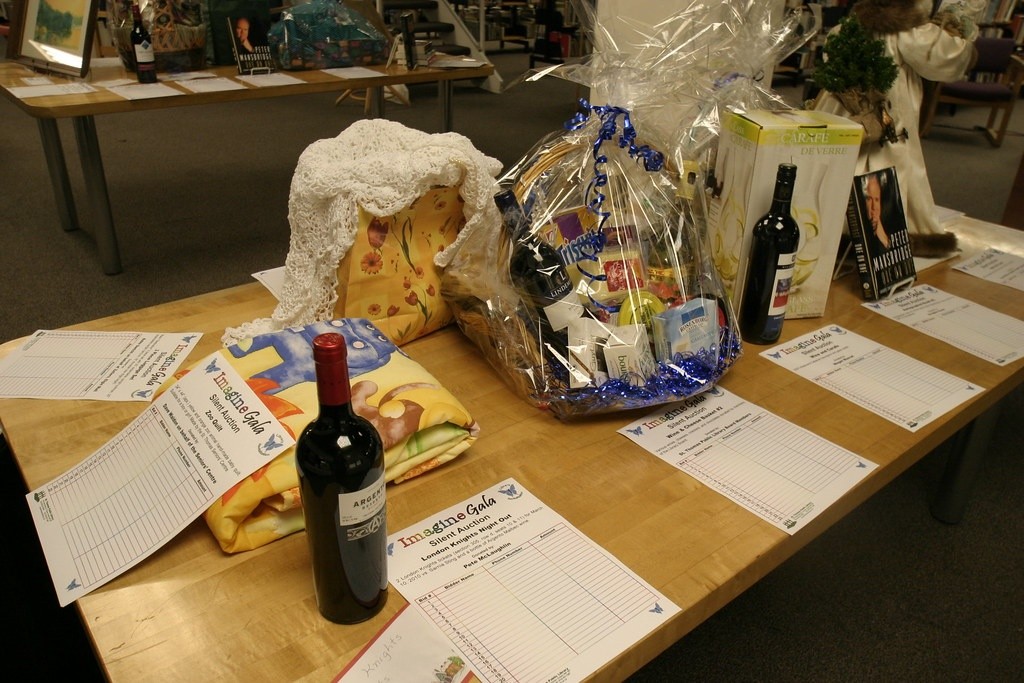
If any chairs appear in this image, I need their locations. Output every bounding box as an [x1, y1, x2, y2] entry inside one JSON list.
[[921, 21, 1024, 147]]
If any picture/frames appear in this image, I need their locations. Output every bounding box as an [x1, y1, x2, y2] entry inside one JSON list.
[[17, 0, 100, 77]]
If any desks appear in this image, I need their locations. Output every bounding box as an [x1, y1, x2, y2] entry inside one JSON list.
[[0, 241, 1024, 683], [0, 55, 496, 277]]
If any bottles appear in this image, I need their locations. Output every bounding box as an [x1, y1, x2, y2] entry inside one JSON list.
[[493, 189, 593, 385], [295, 333, 388, 624], [739, 163, 800, 345], [713, 162, 748, 289], [788, 162, 827, 292], [130, 5, 157, 83], [647, 160, 700, 308]]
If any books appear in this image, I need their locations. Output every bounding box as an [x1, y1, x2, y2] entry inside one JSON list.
[[846, 166, 918, 301], [225, 15, 277, 73], [394, 12, 437, 71]]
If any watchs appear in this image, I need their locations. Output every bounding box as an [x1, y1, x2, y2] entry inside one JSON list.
[[887, 240, 891, 248]]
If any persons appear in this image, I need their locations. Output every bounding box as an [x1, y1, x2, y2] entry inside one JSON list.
[[860, 174, 893, 253], [235, 17, 256, 54]]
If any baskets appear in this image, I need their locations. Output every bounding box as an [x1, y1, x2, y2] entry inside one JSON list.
[[115, 22, 208, 74]]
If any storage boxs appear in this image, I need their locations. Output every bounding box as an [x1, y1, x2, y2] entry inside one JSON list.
[[538, 205, 600, 249], [706, 109, 865, 319], [647, 263, 692, 300], [567, 317, 608, 388], [564, 242, 646, 306], [650, 297, 719, 364], [603, 323, 655, 383]]
